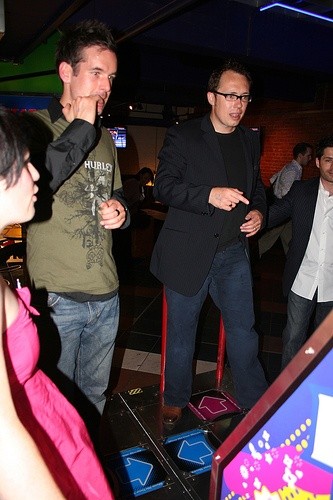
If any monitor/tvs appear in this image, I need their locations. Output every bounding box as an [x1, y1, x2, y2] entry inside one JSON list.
[[104, 124, 128, 149]]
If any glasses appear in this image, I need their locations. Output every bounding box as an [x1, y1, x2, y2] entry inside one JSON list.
[[210, 90, 252, 102]]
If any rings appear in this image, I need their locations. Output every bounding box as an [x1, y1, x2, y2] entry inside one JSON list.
[[229, 204, 232, 207], [115, 209, 120, 216]]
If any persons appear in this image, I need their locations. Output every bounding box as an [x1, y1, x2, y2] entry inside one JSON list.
[[259, 143, 313, 257], [124, 167, 153, 203], [267, 138, 333, 371], [0, 108, 115, 500], [27, 20, 131, 413], [148, 62, 268, 428]]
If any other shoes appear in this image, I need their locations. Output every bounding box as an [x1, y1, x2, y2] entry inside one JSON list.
[[163, 402, 181, 423]]
